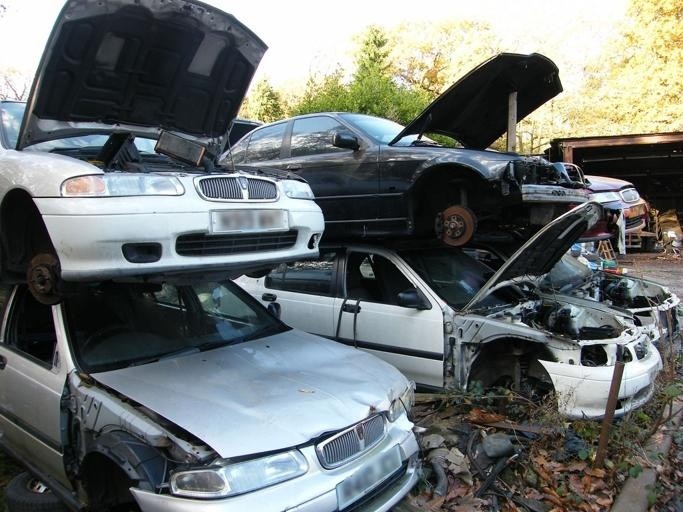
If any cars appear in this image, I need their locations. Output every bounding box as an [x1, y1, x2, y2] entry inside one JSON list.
[[471, 228, 681, 338], [234, 201, 663, 423], [0, 0, 327, 309], [0, 281, 432, 512], [223, 50, 604, 264], [579, 175, 650, 243]]
